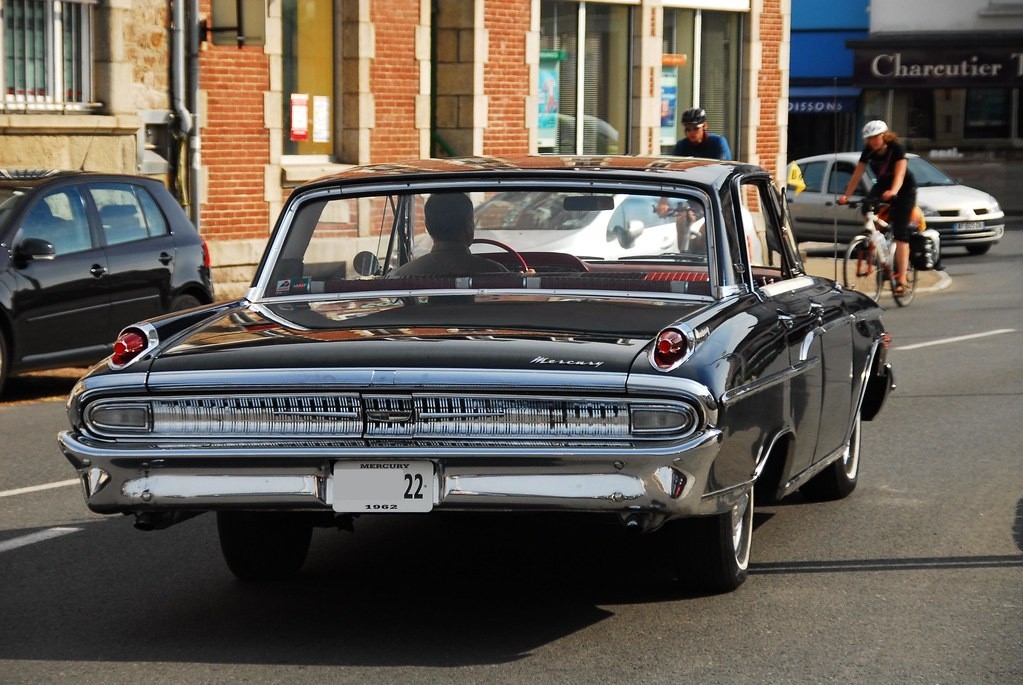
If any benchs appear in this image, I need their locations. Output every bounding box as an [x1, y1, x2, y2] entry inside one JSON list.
[[308, 270, 712, 296]]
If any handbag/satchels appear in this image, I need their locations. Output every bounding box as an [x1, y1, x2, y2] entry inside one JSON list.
[[875, 205, 926, 233], [911, 229, 941, 271]]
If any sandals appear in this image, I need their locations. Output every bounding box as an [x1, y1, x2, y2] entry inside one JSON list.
[[893, 282, 910, 297]]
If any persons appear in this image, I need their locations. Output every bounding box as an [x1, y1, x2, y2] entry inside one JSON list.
[[385, 193, 536, 280], [838, 120, 917, 297], [656, 108, 732, 253]]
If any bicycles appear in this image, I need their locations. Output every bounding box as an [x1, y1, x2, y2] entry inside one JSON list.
[[837, 195, 919, 307]]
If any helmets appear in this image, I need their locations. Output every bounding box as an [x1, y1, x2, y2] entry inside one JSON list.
[[682, 109, 706, 125], [862, 120, 888, 139]]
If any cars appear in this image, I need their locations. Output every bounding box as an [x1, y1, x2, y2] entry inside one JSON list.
[[0, 174, 217, 389], [58, 153, 897, 591], [545, 112, 618, 152], [785, 150, 1005, 256]]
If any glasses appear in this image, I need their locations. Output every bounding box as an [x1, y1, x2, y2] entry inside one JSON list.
[[685, 124, 705, 132]]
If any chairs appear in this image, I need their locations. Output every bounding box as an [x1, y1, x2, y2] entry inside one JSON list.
[[95, 205, 143, 243]]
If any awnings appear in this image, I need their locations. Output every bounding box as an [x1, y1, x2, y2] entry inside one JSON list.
[[789, 85, 866, 115]]
